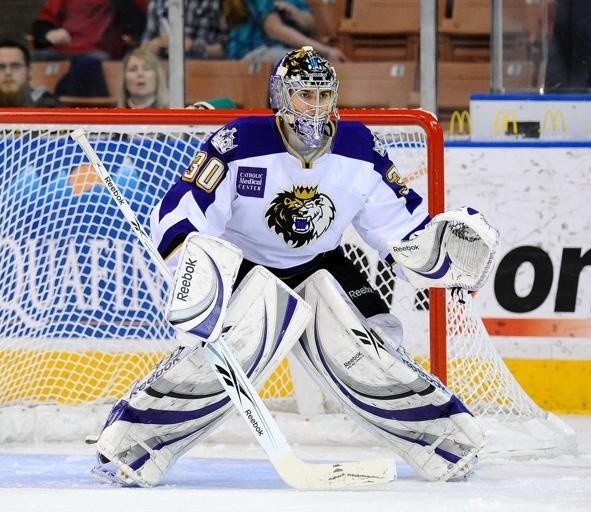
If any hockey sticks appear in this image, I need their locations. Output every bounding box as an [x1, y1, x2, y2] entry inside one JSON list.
[[71, 127, 397, 489]]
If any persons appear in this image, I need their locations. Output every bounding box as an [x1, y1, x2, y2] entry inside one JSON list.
[[82, 43, 503, 485], [112, 46, 169, 109], [542, 3, 591, 99], [140, 2, 229, 62], [225, 1, 352, 65], [1, 37, 71, 109], [23, 1, 145, 99]]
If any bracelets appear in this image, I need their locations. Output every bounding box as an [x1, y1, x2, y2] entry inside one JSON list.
[[190, 38, 209, 60]]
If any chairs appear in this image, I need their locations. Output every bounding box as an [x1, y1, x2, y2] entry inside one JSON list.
[[0, 0, 560, 133]]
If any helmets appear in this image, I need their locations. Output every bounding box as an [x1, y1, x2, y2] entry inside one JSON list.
[[269, 45, 340, 148]]
